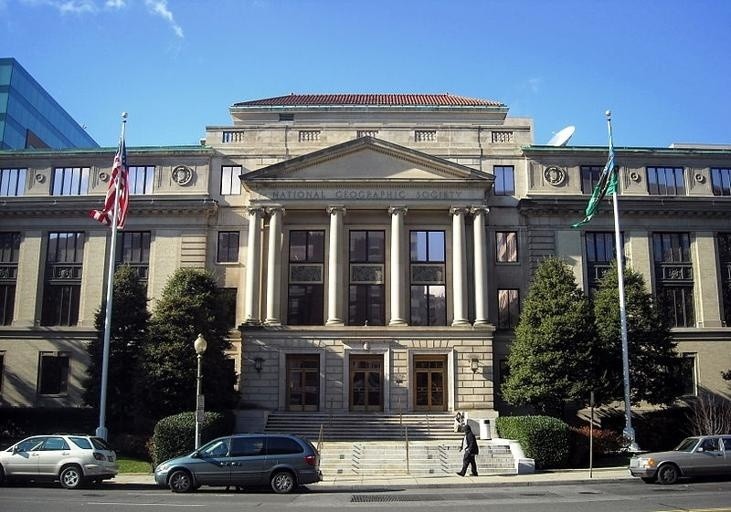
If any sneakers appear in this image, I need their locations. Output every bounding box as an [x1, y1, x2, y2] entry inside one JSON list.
[[469, 473, 479, 476], [455, 471, 464, 477]]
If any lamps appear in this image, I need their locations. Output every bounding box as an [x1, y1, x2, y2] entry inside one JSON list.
[[254, 356, 266, 373], [469, 357, 480, 375]]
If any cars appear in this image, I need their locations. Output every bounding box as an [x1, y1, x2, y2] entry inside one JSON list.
[[625, 434, 731, 486]]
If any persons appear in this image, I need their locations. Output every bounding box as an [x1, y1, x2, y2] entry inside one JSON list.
[[456, 424, 479, 476]]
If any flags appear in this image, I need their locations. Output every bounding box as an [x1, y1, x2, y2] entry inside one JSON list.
[[569, 140, 620, 229], [89, 131, 131, 232]]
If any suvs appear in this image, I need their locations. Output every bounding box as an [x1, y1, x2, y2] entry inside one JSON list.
[[151, 432, 326, 496], [0, 431, 119, 491]]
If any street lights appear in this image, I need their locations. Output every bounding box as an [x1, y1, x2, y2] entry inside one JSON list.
[[192, 331, 208, 452]]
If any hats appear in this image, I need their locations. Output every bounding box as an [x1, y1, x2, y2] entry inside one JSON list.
[[462, 425, 471, 433]]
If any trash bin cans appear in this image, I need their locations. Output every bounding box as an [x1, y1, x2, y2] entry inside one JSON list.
[[479, 419, 492, 440]]
